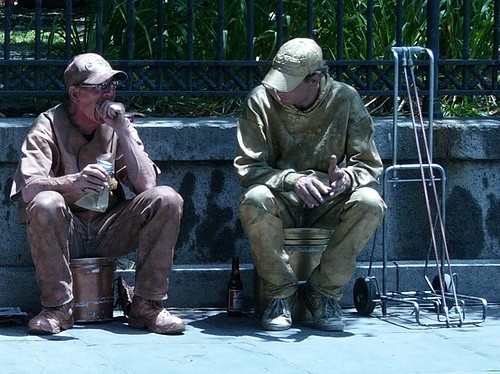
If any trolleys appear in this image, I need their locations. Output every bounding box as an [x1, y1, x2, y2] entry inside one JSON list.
[[354, 46, 489, 329]]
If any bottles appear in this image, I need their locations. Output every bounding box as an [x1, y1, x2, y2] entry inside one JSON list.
[[227, 257, 243, 317]]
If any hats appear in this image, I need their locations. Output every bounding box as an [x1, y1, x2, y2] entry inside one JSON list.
[[261, 38, 323, 92], [63, 53, 128, 86]]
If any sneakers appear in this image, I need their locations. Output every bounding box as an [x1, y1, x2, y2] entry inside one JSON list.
[[262, 296, 292, 330], [129, 296, 186, 334], [28, 303, 74, 335], [299, 283, 345, 331]]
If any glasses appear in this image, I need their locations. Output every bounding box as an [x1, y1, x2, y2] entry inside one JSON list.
[[73, 78, 118, 91]]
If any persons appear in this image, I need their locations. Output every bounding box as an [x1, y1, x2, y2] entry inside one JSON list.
[[233, 38, 388, 331], [10, 53, 186, 336]]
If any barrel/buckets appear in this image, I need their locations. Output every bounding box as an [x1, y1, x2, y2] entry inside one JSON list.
[[258, 227, 336, 319], [69, 257, 118, 322]]
